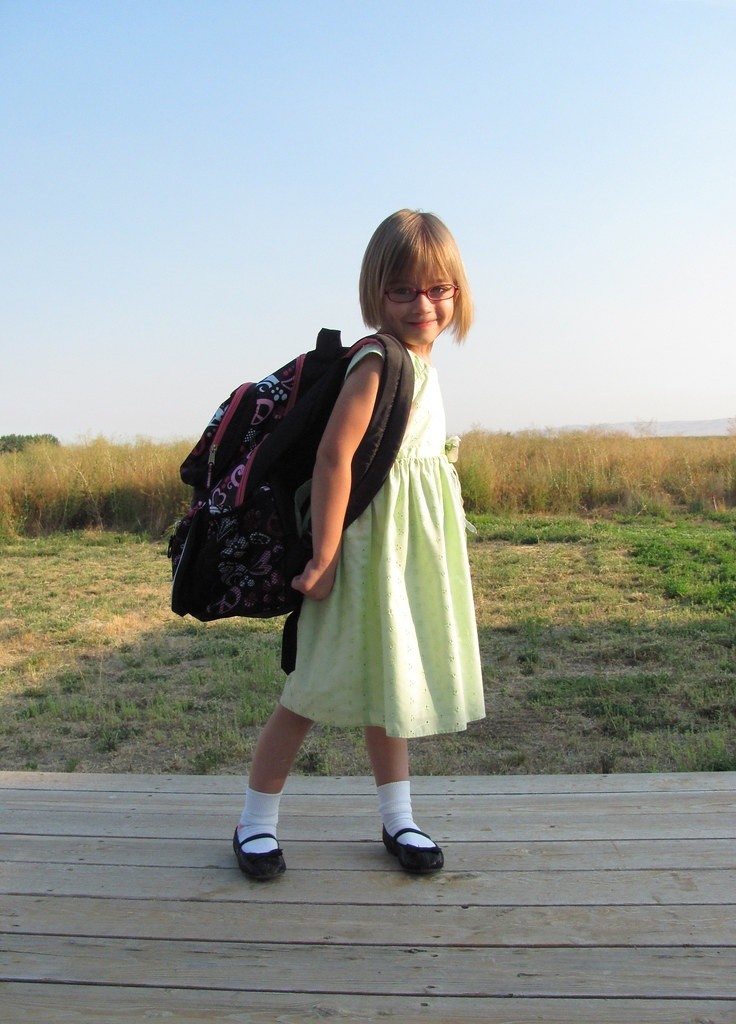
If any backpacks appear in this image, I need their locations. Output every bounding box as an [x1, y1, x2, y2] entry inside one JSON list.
[[168, 328, 415, 676]]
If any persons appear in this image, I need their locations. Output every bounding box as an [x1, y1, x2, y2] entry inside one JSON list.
[[232, 209, 486, 879]]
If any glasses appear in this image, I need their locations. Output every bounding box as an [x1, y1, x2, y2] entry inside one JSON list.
[[384, 282, 459, 303]]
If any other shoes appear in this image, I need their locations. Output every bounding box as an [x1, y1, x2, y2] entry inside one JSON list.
[[232, 825, 286, 879], [382, 822, 445, 871]]
